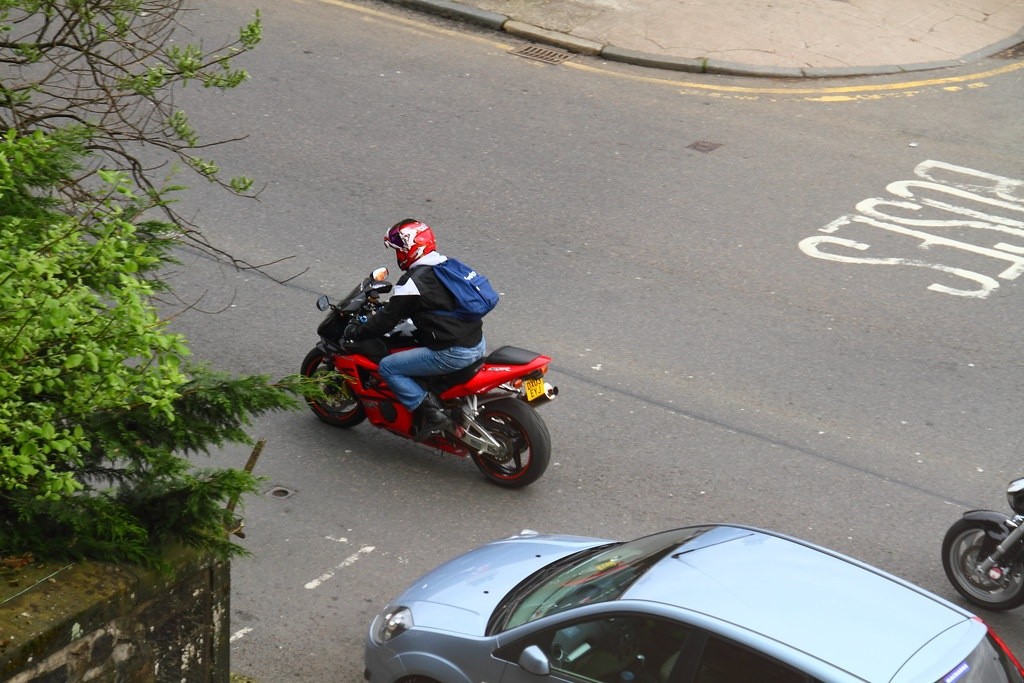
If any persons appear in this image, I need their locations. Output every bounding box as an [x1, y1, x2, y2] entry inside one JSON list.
[[344, 219, 486, 443]]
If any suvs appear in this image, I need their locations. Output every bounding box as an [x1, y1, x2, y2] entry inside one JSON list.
[[363, 520, 1024, 683]]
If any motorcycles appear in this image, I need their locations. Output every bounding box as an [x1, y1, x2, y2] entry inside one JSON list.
[[940, 472, 1024, 610], [300, 267, 558, 488]]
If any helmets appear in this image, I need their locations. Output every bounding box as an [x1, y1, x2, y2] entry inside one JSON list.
[[384, 218, 436, 271]]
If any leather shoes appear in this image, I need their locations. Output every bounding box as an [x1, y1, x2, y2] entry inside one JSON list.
[[413, 394, 453, 443]]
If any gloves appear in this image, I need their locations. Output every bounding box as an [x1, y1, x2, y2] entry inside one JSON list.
[[342, 320, 363, 342]]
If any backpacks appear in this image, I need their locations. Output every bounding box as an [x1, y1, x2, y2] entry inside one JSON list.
[[434, 257, 500, 315]]
